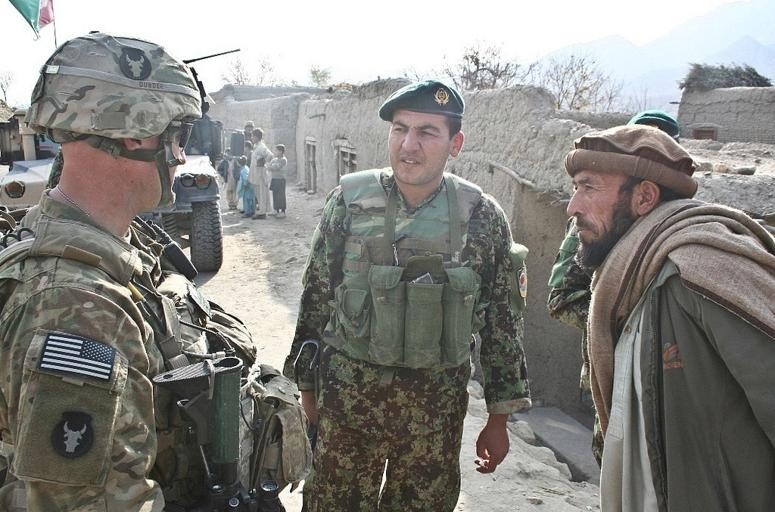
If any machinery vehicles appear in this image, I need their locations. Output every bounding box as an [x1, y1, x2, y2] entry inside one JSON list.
[[2, 108, 247, 275]]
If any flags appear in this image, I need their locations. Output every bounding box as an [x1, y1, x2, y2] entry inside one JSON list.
[[10, 0, 53, 41]]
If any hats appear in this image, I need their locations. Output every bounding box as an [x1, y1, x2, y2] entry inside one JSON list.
[[377, 81, 464, 121], [566, 123, 701, 199]]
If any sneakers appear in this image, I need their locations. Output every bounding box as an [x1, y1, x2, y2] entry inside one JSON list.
[[243, 212, 266, 220], [269, 210, 285, 219], [226, 206, 237, 210]]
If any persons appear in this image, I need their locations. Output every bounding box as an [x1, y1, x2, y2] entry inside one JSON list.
[[545, 108, 680, 471], [282, 78, 533, 512], [0, 29, 316, 512], [565, 122, 775, 512], [217, 121, 289, 220]]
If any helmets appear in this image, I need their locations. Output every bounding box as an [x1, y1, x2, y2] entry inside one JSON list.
[[25, 31, 203, 144], [627, 110, 681, 136], [244, 120, 255, 129]]
